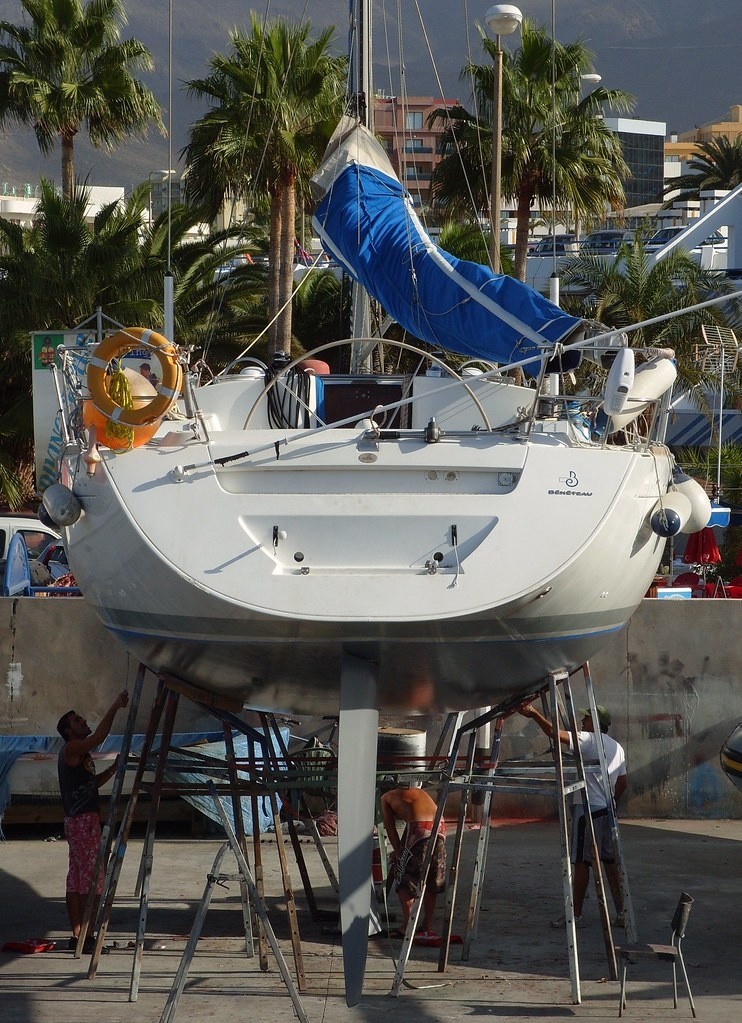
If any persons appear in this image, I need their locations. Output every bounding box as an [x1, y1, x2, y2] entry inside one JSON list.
[[140, 363, 158, 388], [380, 784, 446, 936], [517, 702, 629, 928], [57, 690, 130, 949], [39, 337, 54, 358]]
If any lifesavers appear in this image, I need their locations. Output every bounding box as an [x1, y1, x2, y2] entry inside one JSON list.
[[86, 326, 185, 430]]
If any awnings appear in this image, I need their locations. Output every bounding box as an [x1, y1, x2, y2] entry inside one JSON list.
[[705, 497, 742, 528]]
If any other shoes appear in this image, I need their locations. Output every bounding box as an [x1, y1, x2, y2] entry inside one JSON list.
[[550, 914, 586, 929], [611, 916, 625, 926], [69, 936, 110, 954]]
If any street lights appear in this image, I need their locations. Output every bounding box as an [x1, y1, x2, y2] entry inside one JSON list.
[[485, 1, 525, 284], [570, 61, 602, 255]]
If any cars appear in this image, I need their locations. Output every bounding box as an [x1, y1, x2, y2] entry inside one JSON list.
[[529, 232, 577, 257], [579, 228, 648, 255], [0, 512, 75, 597], [642, 222, 728, 257]]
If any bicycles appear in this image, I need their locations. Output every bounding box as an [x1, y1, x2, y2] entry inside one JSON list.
[[256, 702, 340, 757]]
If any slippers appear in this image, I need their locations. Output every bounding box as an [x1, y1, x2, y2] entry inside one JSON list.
[[415, 927, 426, 933], [384, 929, 407, 937]]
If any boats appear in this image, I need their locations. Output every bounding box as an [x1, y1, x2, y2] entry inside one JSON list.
[[0, 722, 289, 801]]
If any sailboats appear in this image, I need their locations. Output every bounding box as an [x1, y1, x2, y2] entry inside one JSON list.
[[44, 0, 714, 734]]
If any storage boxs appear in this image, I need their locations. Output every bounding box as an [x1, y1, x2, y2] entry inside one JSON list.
[[657, 587, 693, 599]]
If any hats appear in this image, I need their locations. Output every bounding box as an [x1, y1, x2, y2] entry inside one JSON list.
[[579, 706, 612, 727]]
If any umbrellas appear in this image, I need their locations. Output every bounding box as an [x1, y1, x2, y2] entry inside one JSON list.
[[682, 527, 722, 585]]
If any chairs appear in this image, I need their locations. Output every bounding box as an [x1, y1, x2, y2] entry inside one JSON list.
[[615, 891, 697, 1018], [672, 572, 699, 590], [289, 748, 337, 820]]
